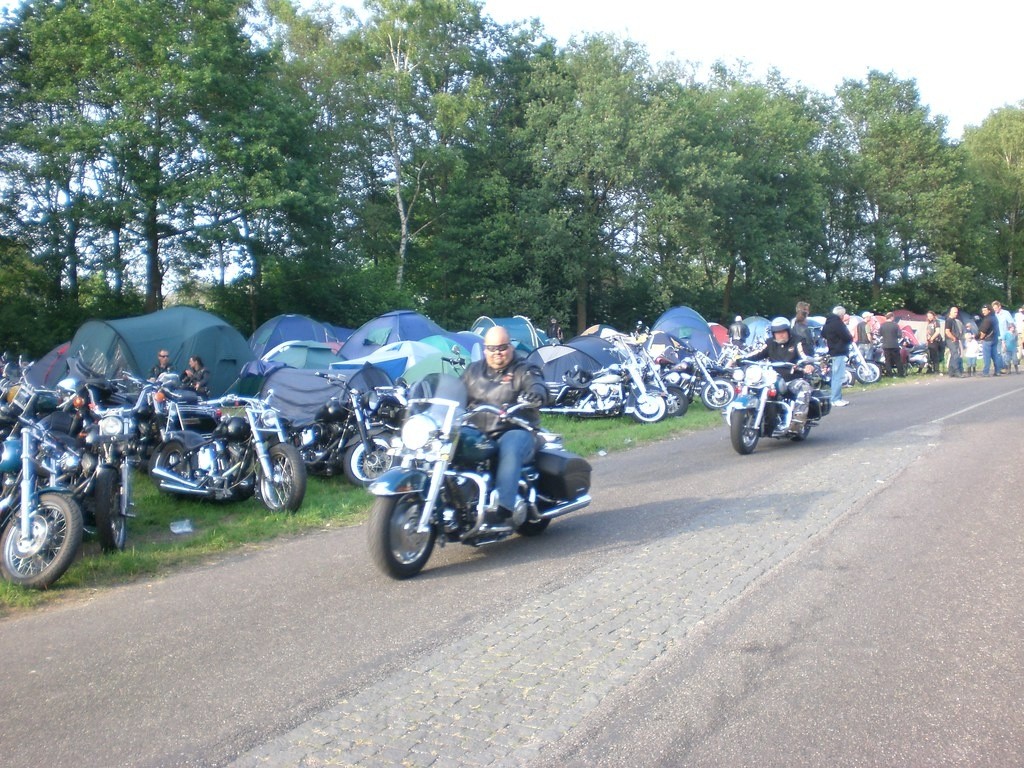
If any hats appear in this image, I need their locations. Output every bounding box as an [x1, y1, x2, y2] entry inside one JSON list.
[[862, 311, 874, 318]]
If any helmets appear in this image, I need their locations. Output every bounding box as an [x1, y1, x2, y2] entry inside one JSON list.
[[771, 317, 791, 339], [735, 316, 742, 322]]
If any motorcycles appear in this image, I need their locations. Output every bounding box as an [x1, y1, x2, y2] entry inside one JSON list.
[[723, 353, 831, 456], [0, 319, 931, 591], [367, 372, 594, 582]]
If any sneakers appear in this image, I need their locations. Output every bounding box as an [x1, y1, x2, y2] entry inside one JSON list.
[[831, 399, 850, 407]]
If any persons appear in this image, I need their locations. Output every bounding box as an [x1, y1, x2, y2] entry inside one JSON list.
[[820, 306, 854, 406], [979, 304, 1001, 376], [843, 314, 858, 343], [878, 311, 914, 378], [944, 305, 980, 378], [790, 302, 814, 359], [727, 315, 750, 350], [857, 311, 875, 361], [547, 316, 564, 344], [458, 326, 545, 525], [926, 311, 943, 372], [149, 348, 181, 382], [736, 316, 814, 432], [179, 353, 211, 397], [991, 301, 1024, 375]]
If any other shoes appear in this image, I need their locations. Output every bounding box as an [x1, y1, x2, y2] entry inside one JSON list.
[[479, 517, 513, 532]]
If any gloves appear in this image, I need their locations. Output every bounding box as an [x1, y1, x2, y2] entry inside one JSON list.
[[519, 391, 545, 404]]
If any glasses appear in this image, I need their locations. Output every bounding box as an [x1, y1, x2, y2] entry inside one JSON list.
[[159, 355, 169, 357], [485, 343, 510, 352]]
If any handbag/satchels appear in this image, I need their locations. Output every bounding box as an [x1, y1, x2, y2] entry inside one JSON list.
[[975, 329, 994, 341]]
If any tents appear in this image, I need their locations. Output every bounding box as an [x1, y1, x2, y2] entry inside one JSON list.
[[339, 307, 725, 405], [789, 310, 978, 343], [711, 316, 774, 349], [23, 306, 359, 404]]
